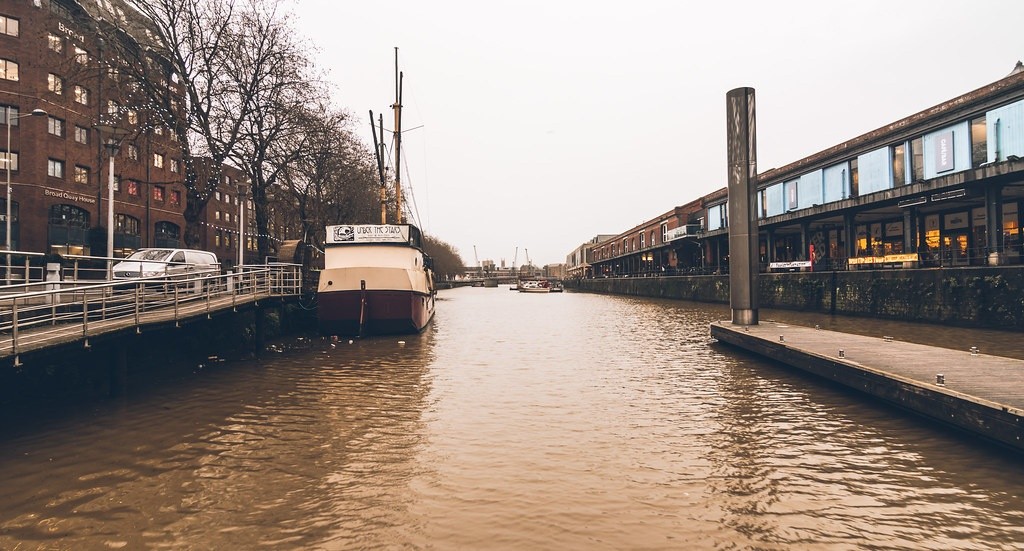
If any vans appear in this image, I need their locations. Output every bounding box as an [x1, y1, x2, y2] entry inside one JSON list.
[[112, 248, 218, 294]]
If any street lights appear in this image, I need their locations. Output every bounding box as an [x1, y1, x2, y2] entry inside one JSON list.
[[233, 181, 251, 294], [6, 109, 47, 285], [92, 124, 134, 296]]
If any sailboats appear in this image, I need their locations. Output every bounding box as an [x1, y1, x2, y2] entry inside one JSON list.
[[314, 47, 437, 336]]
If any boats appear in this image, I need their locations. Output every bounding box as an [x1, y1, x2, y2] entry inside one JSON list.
[[549, 281, 563, 292], [521, 281, 540, 288]]
